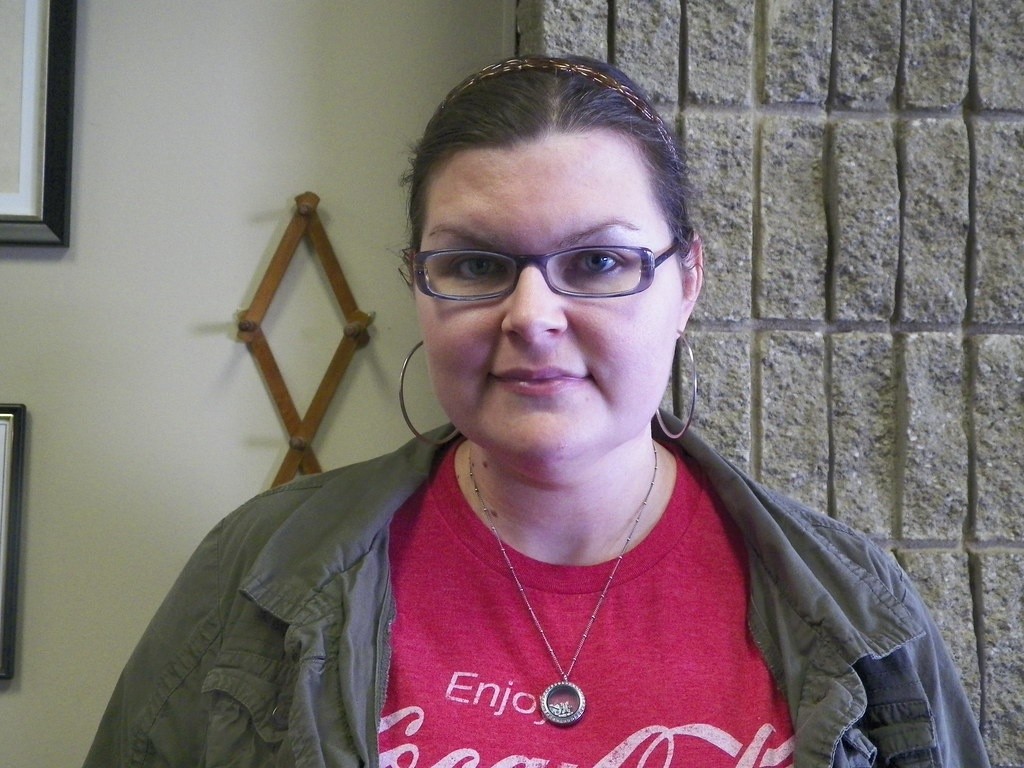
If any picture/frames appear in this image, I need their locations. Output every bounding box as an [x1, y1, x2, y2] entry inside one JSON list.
[[0, 0, 80, 254], [0, 401, 28, 682]]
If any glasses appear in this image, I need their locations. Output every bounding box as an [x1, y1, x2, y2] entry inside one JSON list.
[[410, 239, 685, 301]]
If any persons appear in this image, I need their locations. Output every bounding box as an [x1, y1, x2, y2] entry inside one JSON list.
[[81, 56, 990, 767]]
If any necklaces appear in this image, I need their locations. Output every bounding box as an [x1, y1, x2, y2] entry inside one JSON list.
[[469, 444, 657, 725]]
